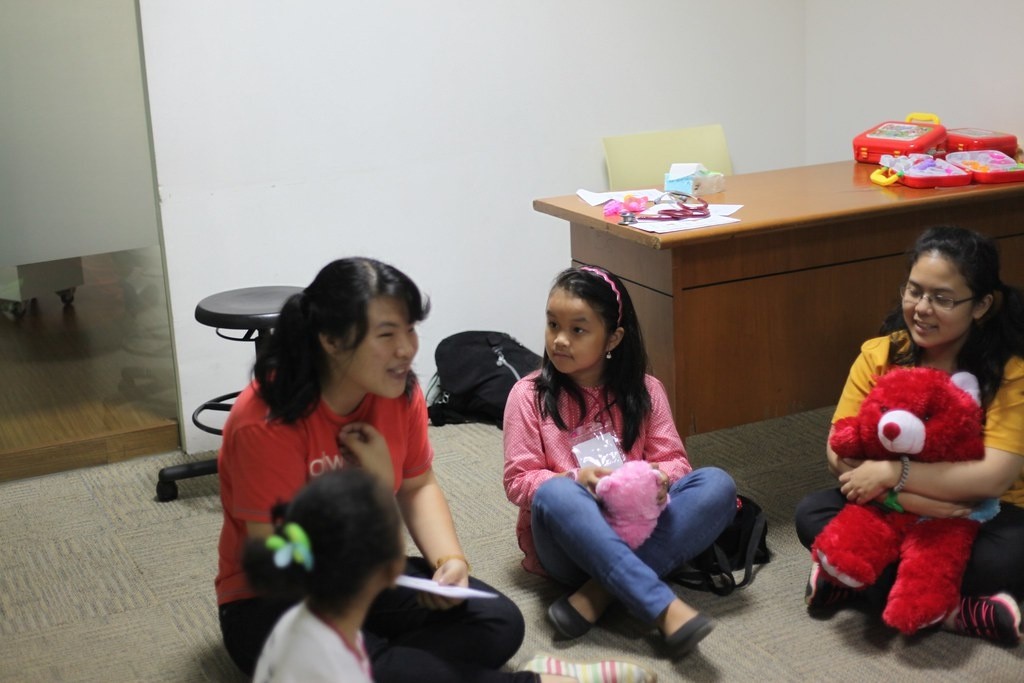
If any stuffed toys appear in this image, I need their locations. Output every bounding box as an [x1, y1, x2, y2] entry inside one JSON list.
[[596, 460, 671, 550], [811, 366, 1001, 636]]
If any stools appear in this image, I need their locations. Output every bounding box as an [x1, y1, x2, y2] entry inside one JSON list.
[[154, 281, 306, 505]]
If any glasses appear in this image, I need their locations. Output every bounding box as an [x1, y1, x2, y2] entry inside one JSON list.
[[898, 279, 977, 310]]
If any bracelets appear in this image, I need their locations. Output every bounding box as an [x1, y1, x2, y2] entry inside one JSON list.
[[876, 491, 904, 513], [894, 456, 911, 494], [433, 555, 472, 572]]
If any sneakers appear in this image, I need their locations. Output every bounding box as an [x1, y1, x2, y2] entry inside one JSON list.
[[955, 593, 1024, 644], [805, 563, 857, 606]]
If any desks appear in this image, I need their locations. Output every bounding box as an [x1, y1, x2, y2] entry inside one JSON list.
[[533, 153, 1024, 450]]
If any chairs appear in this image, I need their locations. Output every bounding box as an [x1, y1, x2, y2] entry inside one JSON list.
[[603, 124, 733, 190]]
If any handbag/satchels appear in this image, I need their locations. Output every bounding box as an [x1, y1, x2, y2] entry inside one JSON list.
[[687, 494, 770, 595], [427, 331, 542, 430]]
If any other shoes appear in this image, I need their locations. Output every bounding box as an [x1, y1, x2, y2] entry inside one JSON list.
[[524, 651, 657, 683], [657, 613, 717, 656], [549, 595, 594, 637]]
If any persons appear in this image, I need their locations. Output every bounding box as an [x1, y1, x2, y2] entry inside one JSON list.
[[503, 267, 737, 660], [214, 257, 659, 683], [795, 227, 1024, 642]]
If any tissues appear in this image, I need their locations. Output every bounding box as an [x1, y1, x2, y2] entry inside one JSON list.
[[664, 162, 728, 197]]
